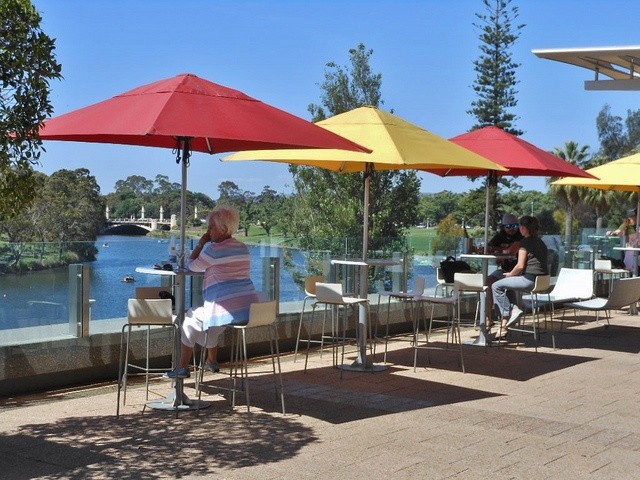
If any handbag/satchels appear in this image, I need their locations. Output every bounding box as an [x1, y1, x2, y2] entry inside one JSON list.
[[441, 256, 472, 283]]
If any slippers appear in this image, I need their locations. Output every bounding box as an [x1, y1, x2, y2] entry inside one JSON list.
[[485, 322, 495, 332]]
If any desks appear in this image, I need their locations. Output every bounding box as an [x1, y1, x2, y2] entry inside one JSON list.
[[460, 251, 515, 347], [330, 257, 400, 372], [135, 263, 210, 410], [613, 247, 640, 316]]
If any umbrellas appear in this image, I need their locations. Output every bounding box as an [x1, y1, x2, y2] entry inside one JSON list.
[[6, 72, 374, 412], [419, 125, 601, 347], [549, 152, 640, 233], [218, 105, 510, 372]]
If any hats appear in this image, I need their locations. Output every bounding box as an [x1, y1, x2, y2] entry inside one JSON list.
[[502, 214, 519, 223]]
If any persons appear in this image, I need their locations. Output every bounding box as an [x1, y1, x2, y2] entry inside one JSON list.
[[607, 208, 640, 273], [166, 206, 253, 380], [487, 213, 524, 327], [491, 215, 547, 338]]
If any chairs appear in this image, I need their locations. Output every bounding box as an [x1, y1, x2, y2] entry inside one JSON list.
[[560, 276, 640, 332], [521, 268, 594, 331]]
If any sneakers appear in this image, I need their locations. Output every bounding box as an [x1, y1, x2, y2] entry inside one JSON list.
[[506, 306, 524, 327], [198, 362, 220, 372], [495, 317, 508, 338], [163, 368, 190, 378]]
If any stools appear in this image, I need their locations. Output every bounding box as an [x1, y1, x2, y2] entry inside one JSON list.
[[411, 284, 466, 375], [136, 287, 199, 401], [116, 298, 182, 419], [594, 259, 631, 317], [374, 277, 432, 366], [294, 276, 359, 364], [453, 273, 493, 353], [428, 266, 480, 341], [304, 282, 374, 376], [497, 275, 556, 352], [255, 291, 270, 303], [195, 300, 287, 425]]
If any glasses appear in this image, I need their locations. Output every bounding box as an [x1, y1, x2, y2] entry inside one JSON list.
[[504, 224, 518, 228], [153, 264, 173, 270]]
